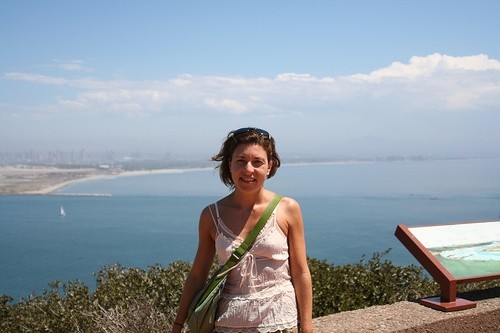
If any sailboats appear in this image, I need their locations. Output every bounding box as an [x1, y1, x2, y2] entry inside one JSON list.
[[58, 204, 66, 217]]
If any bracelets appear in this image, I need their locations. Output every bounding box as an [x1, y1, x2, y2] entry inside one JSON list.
[[174, 322, 184, 329]]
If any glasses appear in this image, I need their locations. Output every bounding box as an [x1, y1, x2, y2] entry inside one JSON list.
[[234, 127, 271, 141]]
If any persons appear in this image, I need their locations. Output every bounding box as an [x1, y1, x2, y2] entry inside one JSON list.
[[171, 128, 313, 333]]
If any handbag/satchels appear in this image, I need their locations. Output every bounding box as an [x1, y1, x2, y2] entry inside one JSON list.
[[181, 269, 227, 333]]
